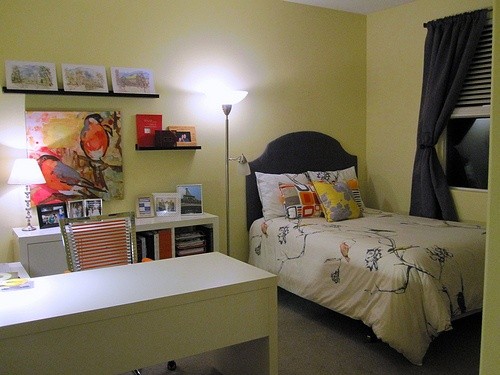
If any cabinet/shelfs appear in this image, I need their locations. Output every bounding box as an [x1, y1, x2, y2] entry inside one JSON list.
[[2, 86, 202, 150], [14, 213, 220, 278]]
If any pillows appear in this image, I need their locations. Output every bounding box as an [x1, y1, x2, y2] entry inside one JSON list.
[[254, 166, 365, 222]]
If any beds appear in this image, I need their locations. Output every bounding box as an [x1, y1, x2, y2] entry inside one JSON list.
[[246, 131, 487, 366]]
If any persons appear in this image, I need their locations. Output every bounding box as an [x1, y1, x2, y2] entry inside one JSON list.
[[159, 200, 174, 211], [72, 205, 81, 217], [44, 214, 58, 224], [179, 133, 188, 142]]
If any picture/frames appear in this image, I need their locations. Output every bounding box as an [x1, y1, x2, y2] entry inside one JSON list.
[[36, 197, 103, 230], [61, 63, 109, 93], [169, 125, 197, 146], [5, 60, 58, 91], [151, 193, 181, 217], [177, 184, 204, 216], [136, 194, 155, 219], [111, 67, 156, 94]]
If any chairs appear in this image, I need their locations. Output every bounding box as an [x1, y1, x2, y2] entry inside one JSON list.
[[58, 211, 178, 375]]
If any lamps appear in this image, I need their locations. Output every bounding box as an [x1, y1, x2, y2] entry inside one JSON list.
[[209, 89, 248, 255], [8, 158, 46, 231]]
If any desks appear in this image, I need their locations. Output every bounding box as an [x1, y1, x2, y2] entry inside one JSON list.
[[0, 251, 279, 375]]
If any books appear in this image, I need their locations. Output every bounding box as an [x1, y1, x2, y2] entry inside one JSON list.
[[136, 114, 162, 146], [137, 231, 160, 262], [176, 232, 206, 256]]
